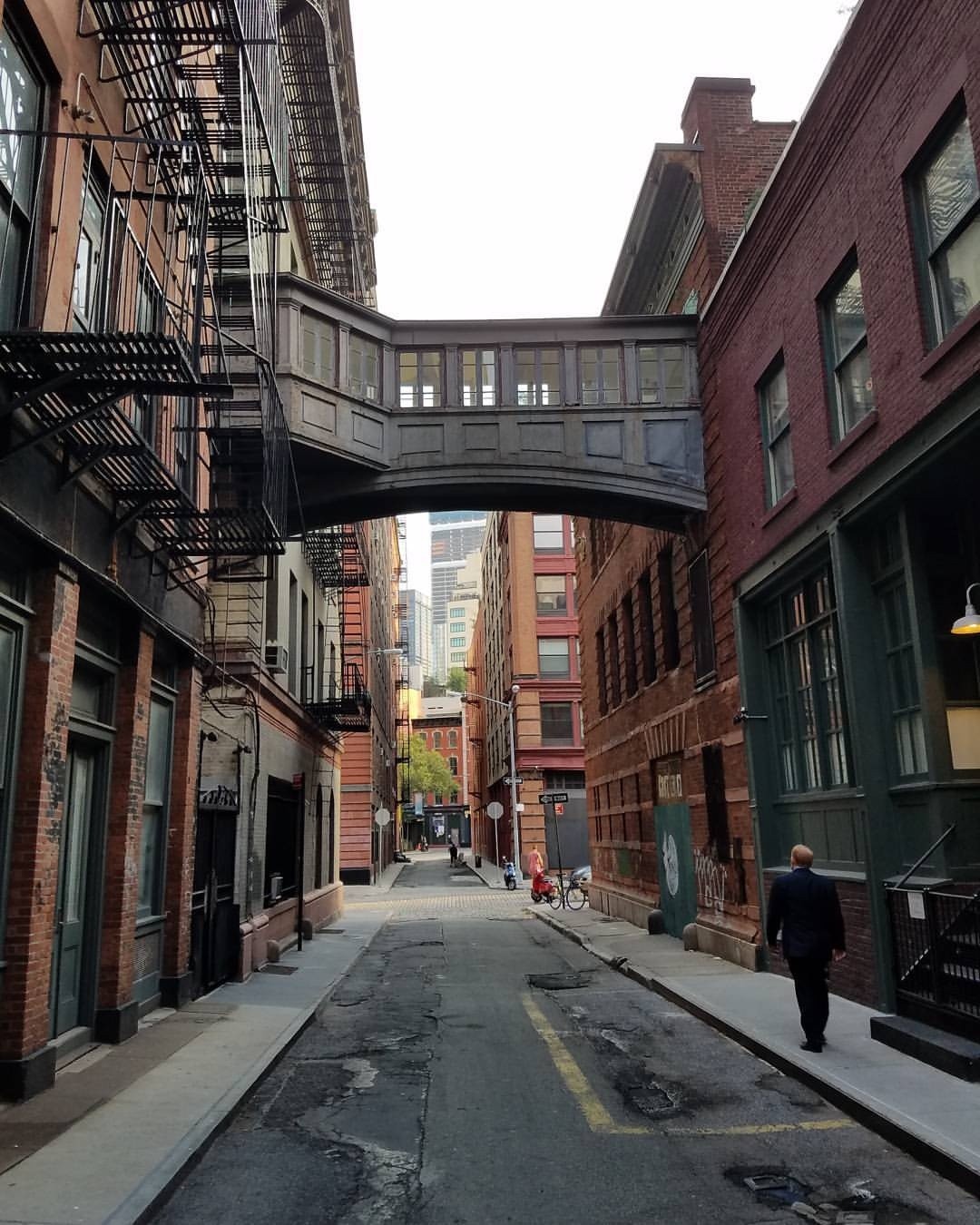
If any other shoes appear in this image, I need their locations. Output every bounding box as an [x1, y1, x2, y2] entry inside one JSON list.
[[802, 1035, 828, 1046], [800, 1041, 822, 1053]]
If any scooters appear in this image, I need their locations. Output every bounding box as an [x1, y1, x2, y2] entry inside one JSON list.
[[502, 856, 517, 891]]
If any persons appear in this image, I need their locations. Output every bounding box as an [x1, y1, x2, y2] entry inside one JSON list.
[[528, 845, 543, 894], [450, 843, 458, 867], [766, 844, 847, 1052]]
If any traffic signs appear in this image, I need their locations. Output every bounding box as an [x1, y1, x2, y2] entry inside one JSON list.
[[503, 776, 523, 785], [537, 792, 569, 805]]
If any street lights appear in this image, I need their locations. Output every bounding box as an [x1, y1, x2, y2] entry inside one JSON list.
[[449, 691, 524, 884]]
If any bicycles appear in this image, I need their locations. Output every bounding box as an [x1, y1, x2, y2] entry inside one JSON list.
[[547, 872, 585, 910]]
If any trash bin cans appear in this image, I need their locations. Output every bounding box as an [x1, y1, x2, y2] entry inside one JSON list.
[[475, 855, 482, 867]]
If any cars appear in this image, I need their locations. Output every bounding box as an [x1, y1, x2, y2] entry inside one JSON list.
[[569, 865, 592, 896]]
